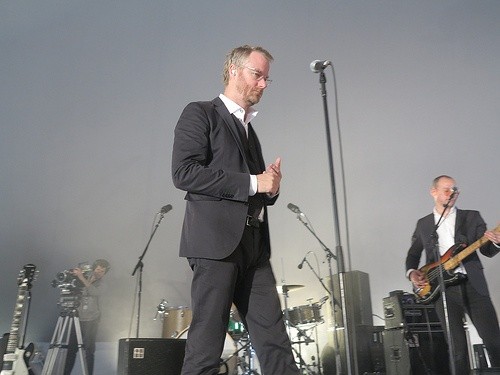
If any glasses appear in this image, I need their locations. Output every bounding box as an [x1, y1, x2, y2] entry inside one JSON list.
[[235, 65, 272, 88]]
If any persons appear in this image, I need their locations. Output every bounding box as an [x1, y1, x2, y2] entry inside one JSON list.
[[63, 259, 110, 375], [171, 44, 301, 375], [404, 175, 500, 375]]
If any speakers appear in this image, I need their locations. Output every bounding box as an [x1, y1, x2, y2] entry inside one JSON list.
[[117, 338, 188, 375], [329, 269, 452, 375]]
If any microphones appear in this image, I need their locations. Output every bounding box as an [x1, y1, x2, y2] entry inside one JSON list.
[[156, 204, 172, 214], [450, 185, 459, 192], [297, 253, 308, 269], [287, 203, 303, 216], [310, 60, 331, 73]]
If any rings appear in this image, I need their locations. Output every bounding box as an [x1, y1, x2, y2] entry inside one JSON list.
[[494, 238, 497, 240]]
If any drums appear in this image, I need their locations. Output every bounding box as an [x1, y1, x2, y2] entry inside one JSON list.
[[175, 323, 238, 375], [230, 303, 243, 323], [282, 303, 325, 329], [161, 305, 194, 339]]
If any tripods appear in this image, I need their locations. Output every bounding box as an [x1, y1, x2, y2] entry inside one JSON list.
[[40, 308, 89, 375]]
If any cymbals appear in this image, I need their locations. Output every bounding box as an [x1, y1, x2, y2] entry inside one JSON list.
[[275, 283, 306, 295]]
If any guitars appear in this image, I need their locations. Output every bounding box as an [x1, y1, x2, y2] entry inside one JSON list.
[[412, 221, 500, 304], [0, 263, 36, 375]]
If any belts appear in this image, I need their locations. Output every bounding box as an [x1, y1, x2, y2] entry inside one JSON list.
[[246, 215, 263, 229]]
